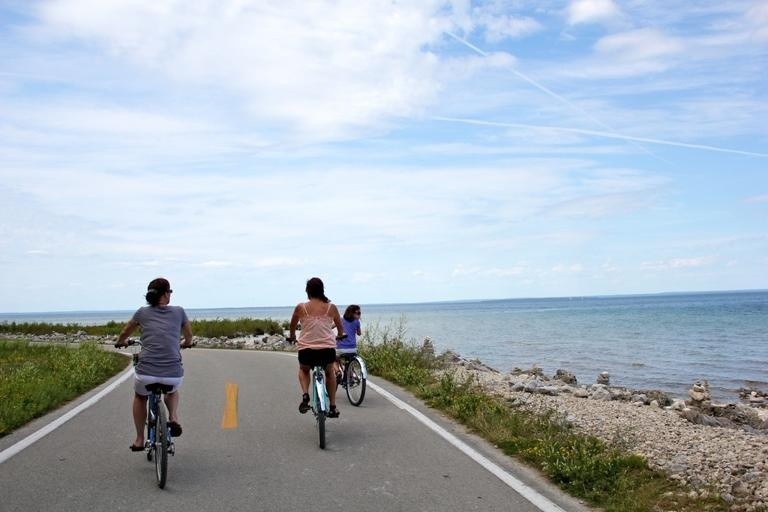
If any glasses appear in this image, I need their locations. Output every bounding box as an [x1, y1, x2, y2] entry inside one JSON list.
[[354, 311, 361, 316], [165, 288, 173, 293]]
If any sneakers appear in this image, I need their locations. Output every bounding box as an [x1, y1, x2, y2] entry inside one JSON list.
[[127, 443, 145, 452], [166, 420, 183, 436]]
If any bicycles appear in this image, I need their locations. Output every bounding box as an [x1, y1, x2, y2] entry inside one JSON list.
[[115, 340, 198, 490], [285, 330, 368, 450]]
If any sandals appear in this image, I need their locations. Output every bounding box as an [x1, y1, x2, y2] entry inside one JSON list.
[[335, 370, 344, 386], [299, 393, 314, 415], [328, 406, 341, 417]]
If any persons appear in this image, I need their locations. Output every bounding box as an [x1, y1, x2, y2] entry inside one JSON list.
[[115, 275, 192, 451], [332, 304, 362, 387], [287, 277, 343, 419]]
